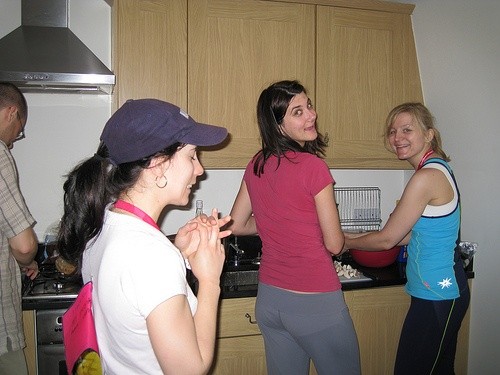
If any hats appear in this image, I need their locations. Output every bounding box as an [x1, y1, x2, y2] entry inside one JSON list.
[[99, 98, 229, 167]]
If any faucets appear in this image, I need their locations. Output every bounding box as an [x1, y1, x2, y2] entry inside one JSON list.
[[229, 234, 244, 265]]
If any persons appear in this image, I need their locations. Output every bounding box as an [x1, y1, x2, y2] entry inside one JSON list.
[[226, 80, 361, 375], [55, 99, 232, 375], [332, 103, 470, 375], [0, 82, 38, 375]]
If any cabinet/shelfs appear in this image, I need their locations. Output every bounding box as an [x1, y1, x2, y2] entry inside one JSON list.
[[205, 286, 470, 375], [110, 0, 426, 169]]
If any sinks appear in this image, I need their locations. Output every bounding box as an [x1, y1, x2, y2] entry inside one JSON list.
[[196, 271, 258, 291]]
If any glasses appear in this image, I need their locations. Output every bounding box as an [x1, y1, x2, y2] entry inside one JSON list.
[[8, 106, 26, 140]]
